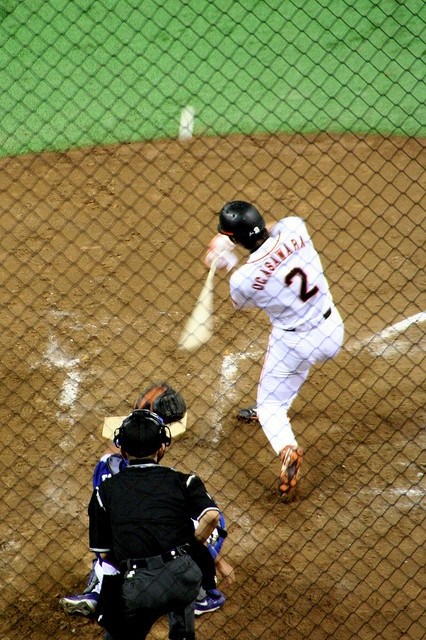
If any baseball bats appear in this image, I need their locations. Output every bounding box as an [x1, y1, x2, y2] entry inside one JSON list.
[[180, 258, 219, 351]]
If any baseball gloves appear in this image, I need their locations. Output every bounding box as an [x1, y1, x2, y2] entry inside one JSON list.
[[132, 384, 187, 425]]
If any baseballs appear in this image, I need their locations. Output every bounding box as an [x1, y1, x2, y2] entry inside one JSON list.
[[177, 105, 195, 139]]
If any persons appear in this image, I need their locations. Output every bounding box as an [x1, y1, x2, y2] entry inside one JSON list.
[[86, 410, 221, 640], [204, 200, 346, 503], [58, 385, 236, 616]]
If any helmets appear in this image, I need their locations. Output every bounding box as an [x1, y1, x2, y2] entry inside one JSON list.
[[114, 410, 172, 464], [137, 383, 186, 436], [218, 201, 263, 242]]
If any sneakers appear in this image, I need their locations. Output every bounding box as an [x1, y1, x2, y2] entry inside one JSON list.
[[235, 407, 261, 422], [194, 589, 224, 617], [59, 595, 96, 613], [279, 444, 302, 503]]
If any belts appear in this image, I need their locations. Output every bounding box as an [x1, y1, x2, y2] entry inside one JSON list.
[[117, 548, 182, 571]]
